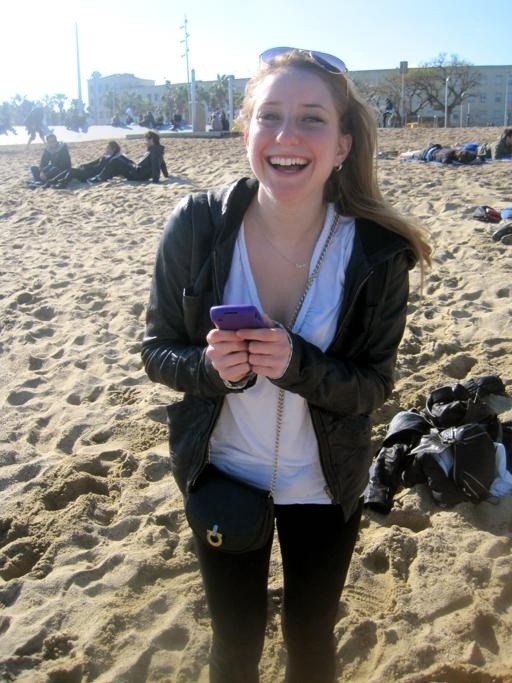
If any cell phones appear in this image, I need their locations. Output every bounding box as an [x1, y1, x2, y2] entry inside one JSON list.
[[209, 306, 267, 330]]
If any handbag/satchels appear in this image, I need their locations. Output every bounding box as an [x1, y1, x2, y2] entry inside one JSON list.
[[185, 463, 275, 555]]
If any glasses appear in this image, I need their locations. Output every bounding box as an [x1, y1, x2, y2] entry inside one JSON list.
[[259, 47, 349, 75]]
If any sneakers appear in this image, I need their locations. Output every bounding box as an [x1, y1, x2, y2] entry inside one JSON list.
[[89, 176, 97, 183]]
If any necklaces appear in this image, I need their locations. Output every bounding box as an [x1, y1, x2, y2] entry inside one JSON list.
[[259, 216, 322, 268]]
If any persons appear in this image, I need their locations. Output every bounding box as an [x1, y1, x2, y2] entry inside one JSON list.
[[401, 129, 512, 164], [140, 46, 431, 683], [0, 108, 183, 144], [31, 130, 168, 188], [208, 106, 229, 132], [383, 97, 393, 127]]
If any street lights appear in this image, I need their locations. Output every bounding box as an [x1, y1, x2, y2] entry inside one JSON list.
[[460, 92, 465, 128], [227, 75, 234, 122], [180, 19, 191, 100], [444, 76, 450, 129]]
